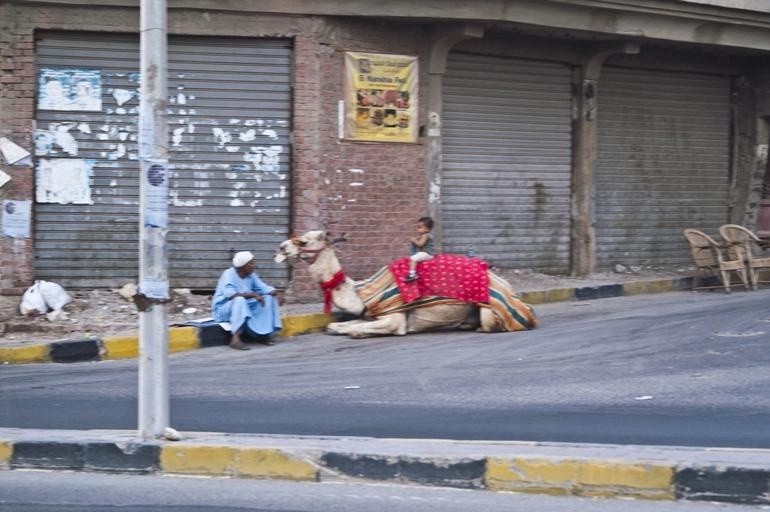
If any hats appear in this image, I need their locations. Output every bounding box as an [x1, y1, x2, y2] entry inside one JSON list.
[[233, 250, 254, 269]]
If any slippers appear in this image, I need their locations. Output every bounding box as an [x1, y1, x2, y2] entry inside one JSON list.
[[229, 341, 250, 350], [261, 338, 275, 346]]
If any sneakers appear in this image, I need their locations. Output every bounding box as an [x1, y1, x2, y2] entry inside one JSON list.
[[403, 272, 419, 281]]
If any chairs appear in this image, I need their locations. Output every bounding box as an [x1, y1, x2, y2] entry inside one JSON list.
[[684, 223, 770, 294]]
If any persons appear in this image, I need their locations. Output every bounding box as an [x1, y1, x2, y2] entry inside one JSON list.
[[210, 248, 281, 352], [400, 218, 437, 281]]
